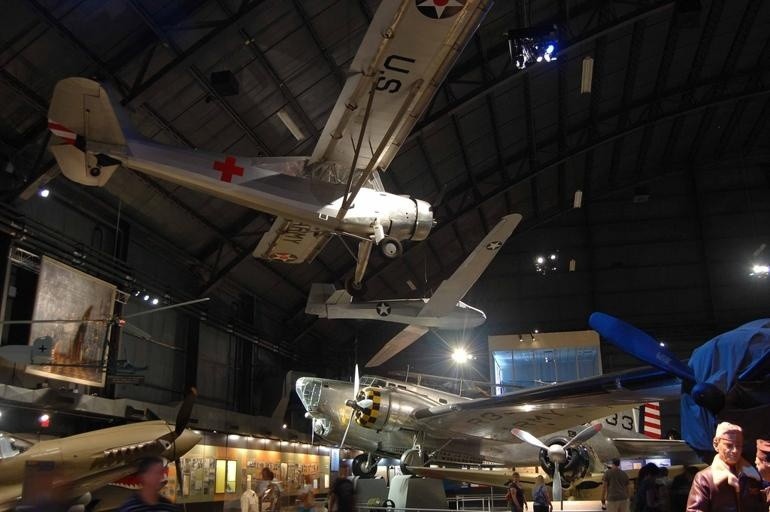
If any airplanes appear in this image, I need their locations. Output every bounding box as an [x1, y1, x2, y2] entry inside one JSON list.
[[0, 387, 204, 511], [292, 355, 700, 512], [405, 420, 714, 504], [306, 209, 524, 368], [21, 0, 492, 292]]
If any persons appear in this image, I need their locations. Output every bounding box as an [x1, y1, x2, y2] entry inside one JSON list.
[[601, 457, 630, 511], [329, 467, 356, 511], [509, 471, 528, 512], [118, 454, 186, 511], [630, 463, 699, 511], [532, 474, 552, 511], [756, 438, 770, 512], [686, 421, 762, 512], [259, 467, 283, 511], [297, 473, 315, 511]]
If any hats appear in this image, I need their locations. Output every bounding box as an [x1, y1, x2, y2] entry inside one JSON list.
[[638, 463, 666, 478], [755, 439, 770, 461]]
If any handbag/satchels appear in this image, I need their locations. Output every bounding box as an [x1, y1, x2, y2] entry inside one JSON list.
[[534, 492, 548, 505], [505, 493, 515, 502]]
